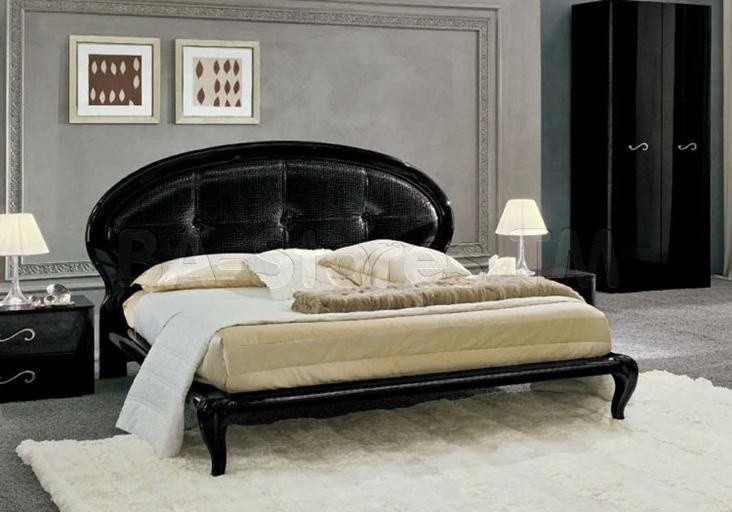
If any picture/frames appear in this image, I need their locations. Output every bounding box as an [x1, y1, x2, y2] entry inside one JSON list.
[[174, 38, 260, 125], [68, 34, 161, 125]]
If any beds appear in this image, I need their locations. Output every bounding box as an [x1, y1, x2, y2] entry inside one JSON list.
[[85, 141, 639, 477]]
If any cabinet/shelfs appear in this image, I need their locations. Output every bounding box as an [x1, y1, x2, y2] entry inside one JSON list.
[[569, 0, 711, 293]]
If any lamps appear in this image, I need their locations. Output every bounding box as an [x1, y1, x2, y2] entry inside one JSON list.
[[495, 199, 549, 276], [0, 213, 50, 307]]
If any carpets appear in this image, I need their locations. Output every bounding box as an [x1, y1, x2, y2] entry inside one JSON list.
[[0, 274, 732, 512], [15, 369, 731, 511]]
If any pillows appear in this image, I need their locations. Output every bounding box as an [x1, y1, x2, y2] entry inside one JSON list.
[[318, 239, 473, 288], [130, 253, 267, 292], [240, 248, 359, 300]]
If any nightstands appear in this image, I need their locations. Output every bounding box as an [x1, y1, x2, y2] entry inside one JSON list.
[[530, 265, 597, 308], [0, 295, 95, 403]]
